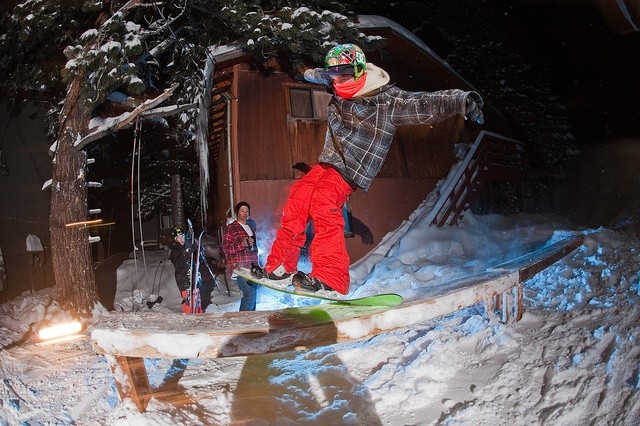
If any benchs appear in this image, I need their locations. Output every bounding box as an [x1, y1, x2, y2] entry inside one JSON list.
[[90, 233, 588, 414]]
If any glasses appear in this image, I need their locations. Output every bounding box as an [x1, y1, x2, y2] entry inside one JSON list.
[[327, 64, 364, 75]]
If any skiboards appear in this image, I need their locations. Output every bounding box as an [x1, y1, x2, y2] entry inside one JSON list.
[[182, 217, 205, 315]]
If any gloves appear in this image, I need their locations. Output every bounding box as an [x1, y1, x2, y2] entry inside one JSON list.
[[464, 94, 486, 124], [295, 73, 309, 84]]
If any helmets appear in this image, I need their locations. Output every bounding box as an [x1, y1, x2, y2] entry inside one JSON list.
[[324, 43, 366, 80], [171, 227, 185, 240]]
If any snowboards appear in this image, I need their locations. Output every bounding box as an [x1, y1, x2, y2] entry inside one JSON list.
[[233, 268, 403, 306]]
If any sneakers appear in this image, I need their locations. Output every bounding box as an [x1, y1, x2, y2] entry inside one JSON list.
[[291, 270, 347, 295], [249, 261, 291, 280]]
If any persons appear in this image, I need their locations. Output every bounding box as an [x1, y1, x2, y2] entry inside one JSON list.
[[170, 228, 202, 313], [222, 201, 259, 313], [251, 43, 485, 296]]
